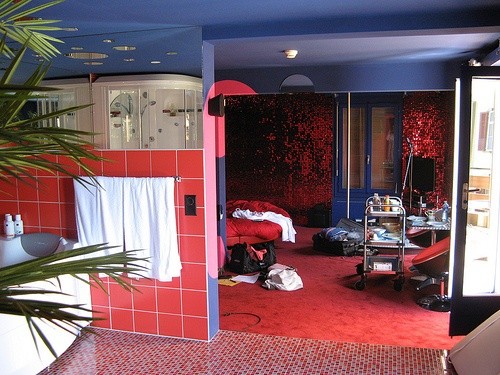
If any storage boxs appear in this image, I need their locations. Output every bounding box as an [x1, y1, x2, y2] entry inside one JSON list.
[[378, 217, 401, 228]]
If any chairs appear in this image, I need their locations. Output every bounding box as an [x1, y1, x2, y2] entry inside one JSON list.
[[412, 236, 453, 312], [406, 228, 450, 272]]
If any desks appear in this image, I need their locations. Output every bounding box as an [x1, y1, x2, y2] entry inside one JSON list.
[[406, 219, 451, 290]]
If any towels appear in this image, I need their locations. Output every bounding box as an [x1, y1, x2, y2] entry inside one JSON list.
[[122, 175, 183, 282], [72, 174, 125, 279]]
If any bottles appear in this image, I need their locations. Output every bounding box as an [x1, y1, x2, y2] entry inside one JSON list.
[[4, 215, 13, 238], [427, 210, 435, 222], [441, 200, 449, 222], [3, 213, 11, 233], [368, 193, 390, 212], [14, 214, 24, 235]]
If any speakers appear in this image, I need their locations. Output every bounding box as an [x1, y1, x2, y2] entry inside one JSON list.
[[406, 156, 435, 190]]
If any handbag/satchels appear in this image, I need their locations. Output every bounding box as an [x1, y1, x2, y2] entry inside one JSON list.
[[262, 263, 304, 292], [231, 243, 276, 272]]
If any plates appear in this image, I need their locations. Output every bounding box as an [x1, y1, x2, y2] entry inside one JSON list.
[[406, 222, 428, 227], [427, 222, 448, 227], [382, 233, 402, 239], [406, 217, 426, 221]]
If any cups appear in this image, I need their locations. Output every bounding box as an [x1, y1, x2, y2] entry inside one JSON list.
[[390, 199, 400, 211], [372, 234, 378, 240]]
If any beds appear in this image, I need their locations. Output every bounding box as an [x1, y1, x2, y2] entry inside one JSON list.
[[225, 200, 293, 264]]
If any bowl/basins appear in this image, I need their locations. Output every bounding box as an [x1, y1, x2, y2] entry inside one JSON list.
[[382, 223, 401, 234], [371, 228, 386, 236]]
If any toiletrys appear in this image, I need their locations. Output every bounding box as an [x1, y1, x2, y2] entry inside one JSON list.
[[2, 213, 23, 239]]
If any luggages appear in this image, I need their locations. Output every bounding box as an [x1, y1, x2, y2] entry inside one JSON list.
[[312, 217, 369, 256]]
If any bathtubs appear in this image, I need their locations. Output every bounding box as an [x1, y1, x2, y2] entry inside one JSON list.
[[1, 231, 94, 375]]
